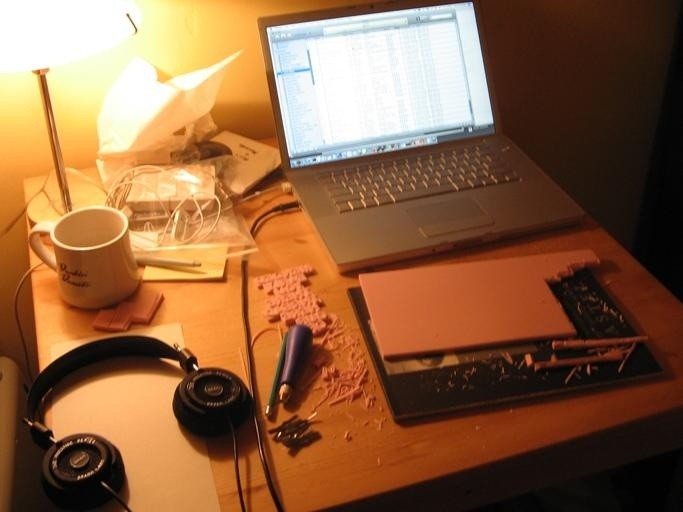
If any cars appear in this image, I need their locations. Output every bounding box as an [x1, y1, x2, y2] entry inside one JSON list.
[[27, 205, 140, 311]]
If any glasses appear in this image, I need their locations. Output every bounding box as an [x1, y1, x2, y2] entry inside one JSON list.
[[0, 1, 138, 219]]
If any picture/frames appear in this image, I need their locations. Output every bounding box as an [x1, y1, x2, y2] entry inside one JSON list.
[[22, 130, 682, 511]]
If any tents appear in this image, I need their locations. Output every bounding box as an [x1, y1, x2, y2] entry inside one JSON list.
[[21, 334, 257, 510]]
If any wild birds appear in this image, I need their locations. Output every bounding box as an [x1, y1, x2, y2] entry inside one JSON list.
[[256, 1, 585, 275]]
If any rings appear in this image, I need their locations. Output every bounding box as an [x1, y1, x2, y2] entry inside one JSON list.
[[265, 332, 290, 416], [138, 255, 202, 267]]
